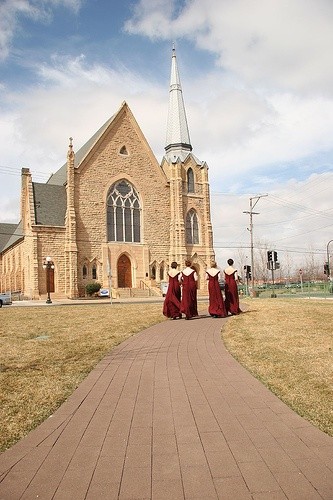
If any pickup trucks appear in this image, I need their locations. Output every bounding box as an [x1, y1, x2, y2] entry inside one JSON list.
[[0, 294, 12, 308]]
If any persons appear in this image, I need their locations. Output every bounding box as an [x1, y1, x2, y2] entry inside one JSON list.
[[179, 260, 199, 320], [223, 258, 242, 315], [163, 262, 183, 320], [205, 261, 227, 317]]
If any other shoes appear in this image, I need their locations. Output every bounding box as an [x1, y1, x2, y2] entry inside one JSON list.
[[213, 315, 217, 317], [178, 317, 183, 319], [173, 317, 177, 320], [185, 317, 189, 320]]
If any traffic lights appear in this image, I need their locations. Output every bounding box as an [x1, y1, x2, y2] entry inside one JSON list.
[[246, 266, 251, 272], [275, 262, 280, 270], [248, 274, 252, 279], [324, 264, 328, 275], [267, 251, 272, 262]]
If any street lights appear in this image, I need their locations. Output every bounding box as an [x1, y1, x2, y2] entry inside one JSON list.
[[43, 256, 54, 303]]
